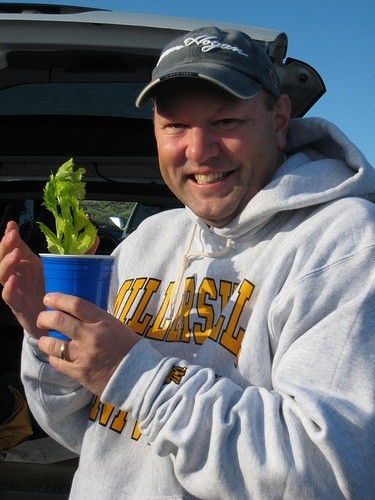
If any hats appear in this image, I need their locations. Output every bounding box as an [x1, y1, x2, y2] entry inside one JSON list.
[[134, 26, 281, 108]]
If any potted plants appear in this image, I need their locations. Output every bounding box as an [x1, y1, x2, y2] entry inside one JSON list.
[[37, 159, 116, 343]]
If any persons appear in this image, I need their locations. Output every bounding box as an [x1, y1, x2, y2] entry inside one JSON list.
[[0, 26, 375, 500]]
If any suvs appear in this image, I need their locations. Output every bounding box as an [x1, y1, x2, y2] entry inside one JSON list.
[[0, 1, 324, 500]]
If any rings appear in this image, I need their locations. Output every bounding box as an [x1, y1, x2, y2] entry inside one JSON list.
[[59, 339, 70, 359]]
